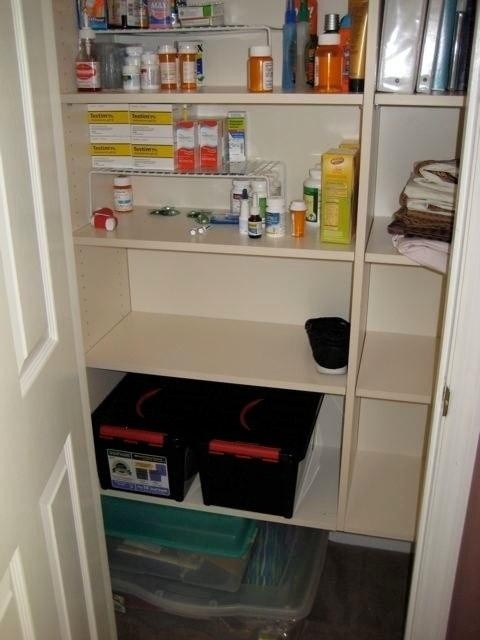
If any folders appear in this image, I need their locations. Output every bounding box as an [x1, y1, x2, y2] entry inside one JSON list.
[[376, 0, 444, 95]]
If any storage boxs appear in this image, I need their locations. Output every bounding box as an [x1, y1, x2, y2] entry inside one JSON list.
[[194, 381, 323, 520], [90, 371, 198, 503], [104, 519, 330, 640], [100, 495, 258, 592]]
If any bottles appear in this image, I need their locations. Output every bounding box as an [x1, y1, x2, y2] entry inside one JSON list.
[[301, 164, 324, 228], [112, 175, 135, 214], [288, 201, 306, 234], [314, 33, 343, 96], [74, 27, 107, 90], [230, 179, 283, 239], [248, 46, 274, 95], [281, 0, 308, 91], [122, 41, 202, 91]]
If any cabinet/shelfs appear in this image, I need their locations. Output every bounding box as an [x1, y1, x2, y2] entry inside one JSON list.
[[47, 1, 478, 551]]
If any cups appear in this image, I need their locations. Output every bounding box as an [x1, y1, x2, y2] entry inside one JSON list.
[[302, 315, 350, 376]]
